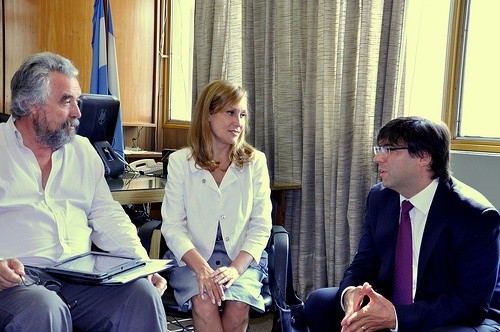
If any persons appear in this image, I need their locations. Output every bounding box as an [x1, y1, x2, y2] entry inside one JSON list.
[[160, 80, 272, 332], [0, 52, 167, 332], [305, 116, 500, 332]]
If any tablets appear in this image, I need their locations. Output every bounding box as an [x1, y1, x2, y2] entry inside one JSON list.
[[46, 250, 141, 277]]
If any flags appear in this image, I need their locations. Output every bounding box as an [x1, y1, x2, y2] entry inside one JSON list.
[[90, 0, 126, 159]]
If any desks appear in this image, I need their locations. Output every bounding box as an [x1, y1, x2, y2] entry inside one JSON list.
[[106, 166, 301, 237]]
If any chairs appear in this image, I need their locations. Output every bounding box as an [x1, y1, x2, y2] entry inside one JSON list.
[[138, 215, 288, 332]]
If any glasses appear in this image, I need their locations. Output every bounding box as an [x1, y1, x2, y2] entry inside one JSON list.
[[19, 268, 78, 312], [373, 146, 408, 157]]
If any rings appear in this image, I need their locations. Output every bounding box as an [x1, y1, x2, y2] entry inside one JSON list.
[[361, 326, 366, 331]]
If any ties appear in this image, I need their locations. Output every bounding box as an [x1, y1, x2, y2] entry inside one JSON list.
[[392, 200, 415, 305]]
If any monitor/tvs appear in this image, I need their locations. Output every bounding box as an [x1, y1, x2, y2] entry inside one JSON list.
[[75, 93, 125, 178]]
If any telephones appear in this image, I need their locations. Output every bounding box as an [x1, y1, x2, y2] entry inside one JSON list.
[[126, 159, 163, 175]]
